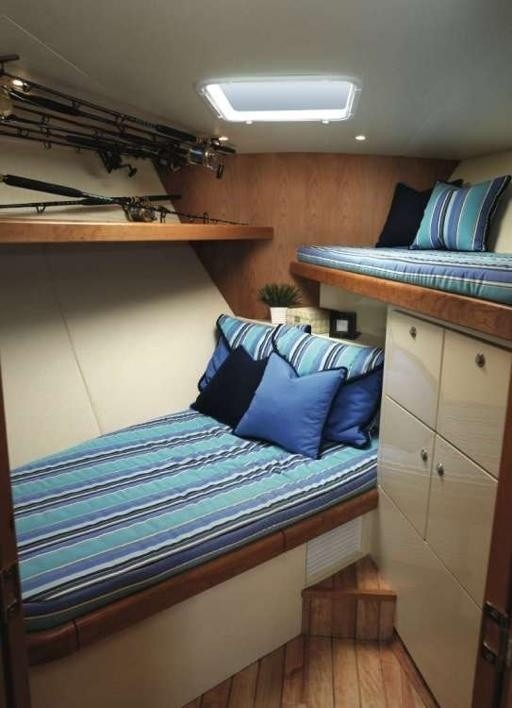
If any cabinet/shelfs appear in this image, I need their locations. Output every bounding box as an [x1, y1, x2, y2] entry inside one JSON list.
[[373, 304, 512, 707]]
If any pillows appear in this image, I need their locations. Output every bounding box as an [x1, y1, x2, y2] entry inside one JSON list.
[[190, 314, 386, 460], [376, 171, 512, 252]]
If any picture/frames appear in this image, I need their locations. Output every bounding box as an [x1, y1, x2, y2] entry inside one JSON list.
[[329, 310, 356, 339]]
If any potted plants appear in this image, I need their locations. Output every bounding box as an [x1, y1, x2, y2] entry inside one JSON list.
[[256, 280, 304, 325]]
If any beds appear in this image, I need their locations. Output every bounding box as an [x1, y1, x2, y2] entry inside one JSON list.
[[8, 314, 382, 705]]
